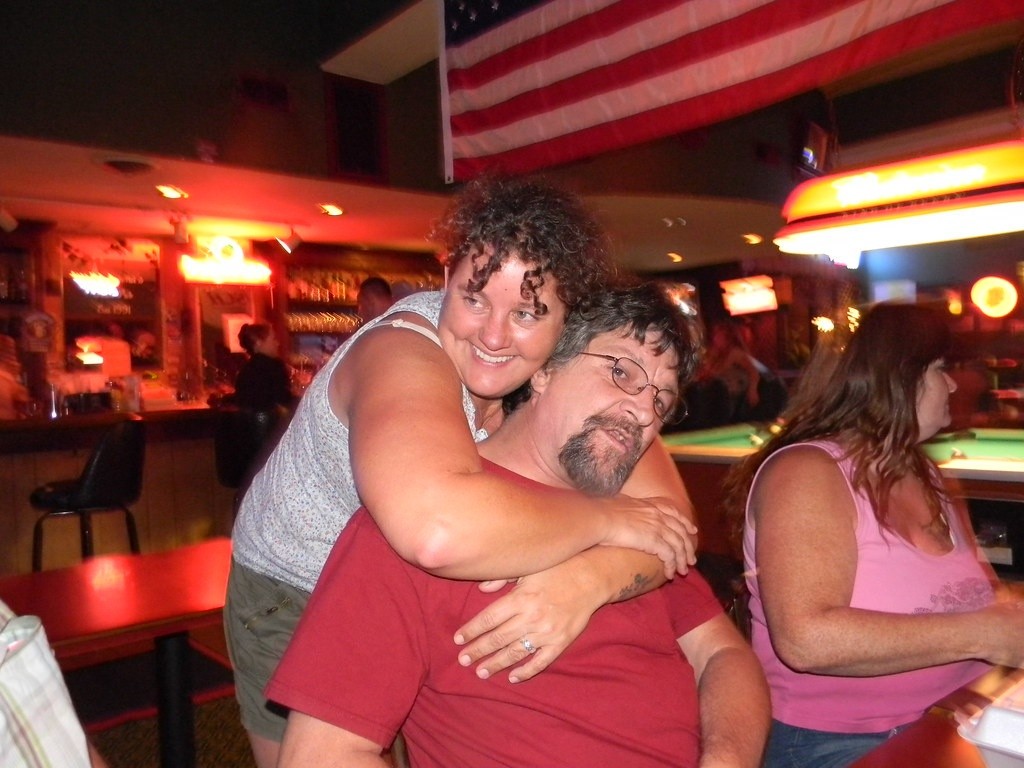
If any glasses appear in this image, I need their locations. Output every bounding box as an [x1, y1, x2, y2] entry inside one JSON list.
[[573, 348, 689, 427]]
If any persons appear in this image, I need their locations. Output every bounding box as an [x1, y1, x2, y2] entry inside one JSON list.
[[258, 279, 1024, 768], [221, 176, 699, 768], [0, 599, 108, 768]]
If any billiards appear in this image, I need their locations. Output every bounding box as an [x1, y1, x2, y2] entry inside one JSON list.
[[751, 437, 763, 446], [749, 433, 757, 441], [770, 424, 780, 434]]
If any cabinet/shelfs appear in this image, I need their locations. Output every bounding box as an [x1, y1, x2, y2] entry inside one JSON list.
[[271, 241, 444, 401]]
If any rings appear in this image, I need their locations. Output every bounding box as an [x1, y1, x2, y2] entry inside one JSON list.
[[520, 639, 532, 651]]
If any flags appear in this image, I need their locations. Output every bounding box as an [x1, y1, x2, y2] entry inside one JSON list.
[[433, 0, 1024, 184]]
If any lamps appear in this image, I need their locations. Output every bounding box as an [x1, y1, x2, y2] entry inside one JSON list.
[[774, 139, 1024, 257]]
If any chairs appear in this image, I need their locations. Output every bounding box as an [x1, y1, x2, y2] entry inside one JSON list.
[[28, 420, 146, 572], [213, 394, 278, 523]]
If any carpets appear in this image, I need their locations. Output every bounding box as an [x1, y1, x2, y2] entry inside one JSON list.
[[59, 639, 235, 734]]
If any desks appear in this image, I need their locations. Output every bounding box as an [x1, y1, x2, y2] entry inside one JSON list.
[[0, 538, 232, 768], [850, 661, 1024, 768], [660, 425, 1024, 585]]
